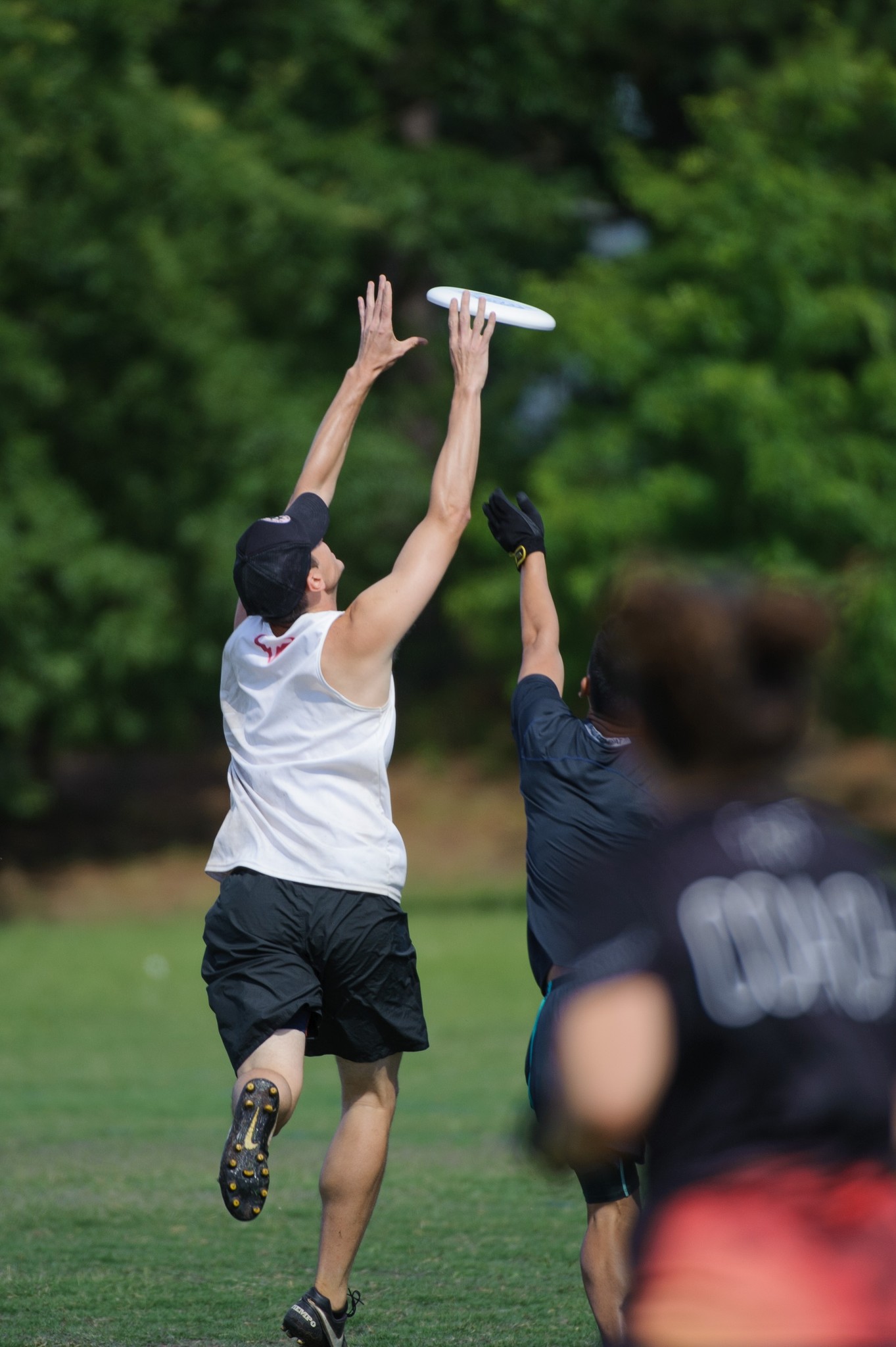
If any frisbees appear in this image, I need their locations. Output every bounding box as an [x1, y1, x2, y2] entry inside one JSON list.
[[425, 286, 556, 331]]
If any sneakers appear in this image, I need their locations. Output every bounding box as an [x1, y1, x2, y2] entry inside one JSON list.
[[218, 1078, 279, 1222], [280, 1287, 365, 1347]]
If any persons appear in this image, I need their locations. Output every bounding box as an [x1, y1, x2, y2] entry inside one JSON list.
[[199, 272, 497, 1347], [482, 488, 678, 1347], [520, 557, 896, 1347]]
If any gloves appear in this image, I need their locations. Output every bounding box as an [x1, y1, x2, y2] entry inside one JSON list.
[[483, 487, 546, 573]]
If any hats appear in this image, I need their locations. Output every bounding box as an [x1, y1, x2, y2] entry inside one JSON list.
[[233, 492, 330, 622]]
